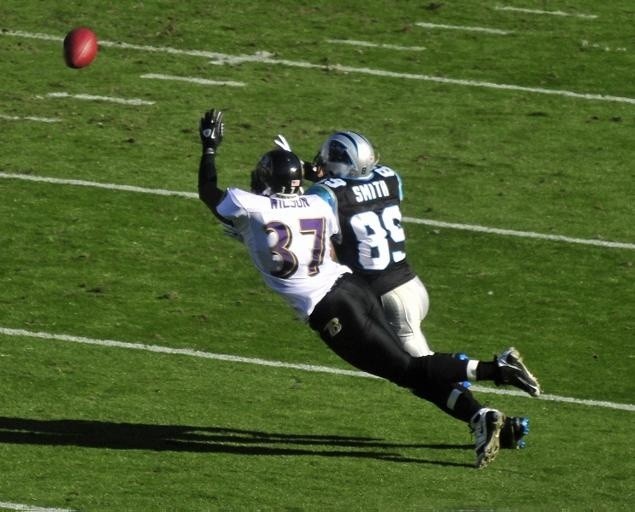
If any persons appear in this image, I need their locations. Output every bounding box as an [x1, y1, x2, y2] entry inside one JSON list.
[[219, 129, 531, 451], [196, 107, 543, 470]]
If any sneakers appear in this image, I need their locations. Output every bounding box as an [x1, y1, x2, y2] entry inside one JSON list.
[[451, 352, 471, 388], [469, 407, 529, 470], [494, 347, 540, 397]]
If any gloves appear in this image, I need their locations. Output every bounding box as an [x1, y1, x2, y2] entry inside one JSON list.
[[199, 108, 224, 155], [222, 224, 243, 241], [274, 134, 316, 180]]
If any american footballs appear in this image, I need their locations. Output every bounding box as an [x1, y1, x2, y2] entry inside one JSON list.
[[63, 26, 98, 69]]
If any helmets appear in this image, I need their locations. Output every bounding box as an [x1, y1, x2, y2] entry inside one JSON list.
[[319, 130, 375, 178], [251, 150, 302, 194]]
